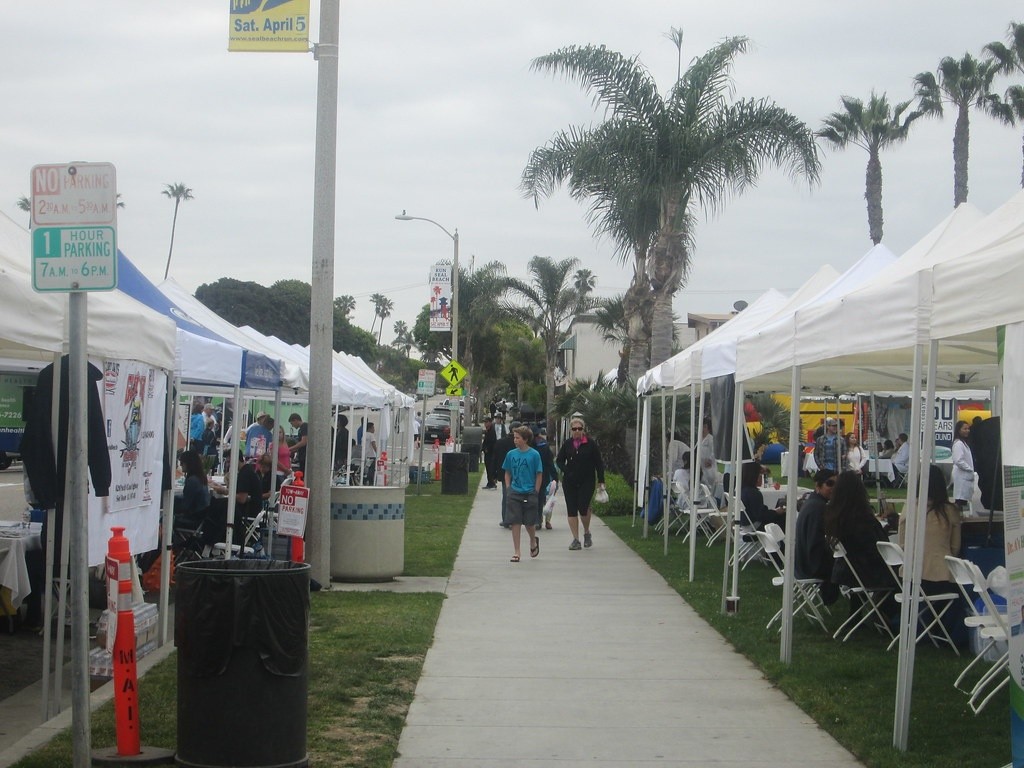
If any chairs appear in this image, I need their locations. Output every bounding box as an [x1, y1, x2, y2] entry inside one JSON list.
[[39, 574, 71, 636], [648, 469, 1009, 715], [173, 458, 368, 569]]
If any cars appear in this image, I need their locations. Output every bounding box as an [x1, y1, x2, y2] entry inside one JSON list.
[[428, 396, 477, 432], [331, 405, 380, 414], [424, 417, 451, 443], [406, 387, 444, 402], [495, 401, 513, 413]]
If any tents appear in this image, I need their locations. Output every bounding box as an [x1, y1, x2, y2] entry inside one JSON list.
[[631, 184, 1024, 753], [0, 199, 415, 726]]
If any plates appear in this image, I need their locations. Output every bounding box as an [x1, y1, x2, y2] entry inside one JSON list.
[[0, 520, 20, 528]]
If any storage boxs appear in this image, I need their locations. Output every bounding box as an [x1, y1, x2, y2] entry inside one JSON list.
[[413, 470, 430, 484]]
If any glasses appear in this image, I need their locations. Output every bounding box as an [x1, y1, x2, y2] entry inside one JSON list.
[[823, 480, 835, 487], [571, 427, 583, 431]]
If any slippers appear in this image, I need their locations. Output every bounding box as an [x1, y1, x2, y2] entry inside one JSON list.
[[510, 555, 520, 562], [530, 537, 539, 558]]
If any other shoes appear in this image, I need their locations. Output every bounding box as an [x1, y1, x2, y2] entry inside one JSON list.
[[482, 483, 497, 490], [535, 524, 542, 530], [24, 608, 41, 631], [569, 539, 582, 550], [546, 522, 552, 529], [584, 533, 591, 547]]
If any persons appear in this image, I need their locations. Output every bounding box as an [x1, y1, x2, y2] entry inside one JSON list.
[[892, 433, 909, 488], [173, 450, 211, 523], [288, 413, 307, 472], [333, 414, 349, 473], [351, 439, 361, 460], [482, 413, 558, 530], [269, 425, 290, 470], [243, 417, 274, 461], [845, 432, 867, 477], [362, 422, 377, 486], [414, 411, 422, 449], [501, 401, 507, 421], [814, 420, 847, 470], [501, 426, 542, 562], [202, 418, 220, 476], [950, 420, 974, 510], [897, 464, 962, 647], [357, 417, 368, 445], [739, 462, 786, 550], [824, 470, 903, 629], [489, 401, 496, 418], [556, 418, 605, 550], [794, 469, 864, 625]]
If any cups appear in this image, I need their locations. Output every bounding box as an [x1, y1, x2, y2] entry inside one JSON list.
[[767, 477, 772, 484]]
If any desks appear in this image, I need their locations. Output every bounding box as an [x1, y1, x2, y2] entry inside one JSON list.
[[0, 519, 43, 634], [946, 546, 1006, 644], [859, 459, 896, 486], [174, 475, 224, 504]]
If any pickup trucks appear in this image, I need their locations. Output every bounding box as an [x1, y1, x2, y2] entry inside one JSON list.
[[0, 426, 26, 472]]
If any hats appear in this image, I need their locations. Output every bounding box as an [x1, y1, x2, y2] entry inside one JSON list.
[[257, 411, 266, 419], [203, 403, 215, 410], [529, 425, 541, 435], [492, 413, 501, 418], [826, 417, 837, 425], [482, 417, 492, 422]]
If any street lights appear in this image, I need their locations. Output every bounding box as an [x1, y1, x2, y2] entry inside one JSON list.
[[396, 215, 458, 447]]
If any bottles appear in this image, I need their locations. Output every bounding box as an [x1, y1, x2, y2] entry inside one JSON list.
[[22, 508, 31, 529]]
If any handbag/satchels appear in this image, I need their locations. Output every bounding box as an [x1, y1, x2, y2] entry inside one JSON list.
[[640, 480, 664, 526], [542, 482, 558, 515], [595, 488, 610, 503], [143, 551, 175, 589]]
